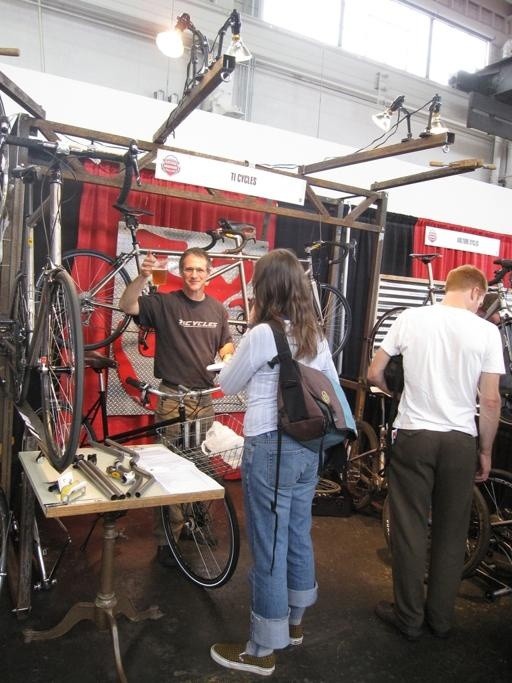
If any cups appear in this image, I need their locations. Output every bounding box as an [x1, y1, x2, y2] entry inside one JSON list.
[[151, 260, 169, 285]]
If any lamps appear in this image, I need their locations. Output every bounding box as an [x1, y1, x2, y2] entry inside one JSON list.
[[154, 9, 254, 64], [368, 94, 448, 140]]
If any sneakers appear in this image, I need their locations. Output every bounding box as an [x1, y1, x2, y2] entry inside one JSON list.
[[288, 623, 305, 645], [209, 639, 276, 676]]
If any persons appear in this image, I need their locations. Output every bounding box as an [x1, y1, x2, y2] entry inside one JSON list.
[[118, 247, 234, 568], [210, 247, 341, 676], [367, 264, 507, 643]]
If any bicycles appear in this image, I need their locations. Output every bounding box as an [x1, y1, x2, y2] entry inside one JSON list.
[[19, 350, 240, 588], [342, 385, 491, 585], [368, 252, 512, 427]]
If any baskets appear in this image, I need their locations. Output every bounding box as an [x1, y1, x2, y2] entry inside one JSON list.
[[155, 412, 246, 479]]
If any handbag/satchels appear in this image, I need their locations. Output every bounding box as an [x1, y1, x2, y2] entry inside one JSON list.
[[276, 360, 358, 454]]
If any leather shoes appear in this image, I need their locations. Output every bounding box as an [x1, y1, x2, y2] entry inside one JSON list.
[[426, 618, 450, 639], [375, 599, 421, 639], [157, 544, 178, 568], [182, 526, 219, 545]]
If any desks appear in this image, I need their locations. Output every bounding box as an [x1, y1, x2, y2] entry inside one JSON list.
[[11, 444, 225, 682]]
